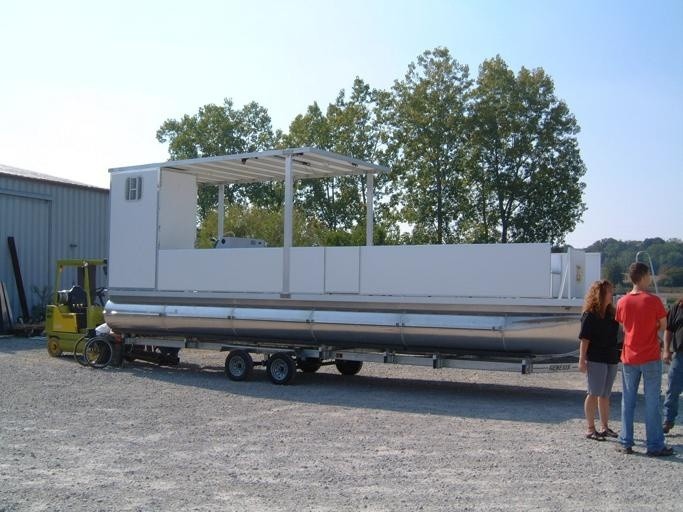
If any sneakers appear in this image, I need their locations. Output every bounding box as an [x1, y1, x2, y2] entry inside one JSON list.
[[663, 420, 674, 432], [648, 447, 673, 456], [623, 448, 632, 454], [586, 431, 605, 440], [601, 429, 617, 437]]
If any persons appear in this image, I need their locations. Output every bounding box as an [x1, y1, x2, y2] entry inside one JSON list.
[[614, 262, 674, 457], [577, 280, 619, 441], [662, 299, 683, 433]]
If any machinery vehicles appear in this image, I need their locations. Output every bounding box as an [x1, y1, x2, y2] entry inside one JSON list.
[[43, 258, 181, 367]]
